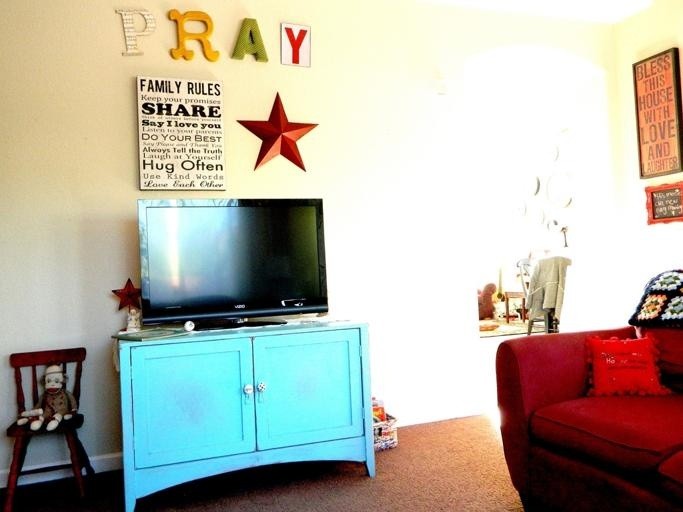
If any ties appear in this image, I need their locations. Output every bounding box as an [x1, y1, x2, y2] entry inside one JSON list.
[[137, 197, 330, 332]]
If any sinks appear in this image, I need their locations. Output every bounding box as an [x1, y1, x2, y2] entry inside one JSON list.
[[497, 326, 683, 512]]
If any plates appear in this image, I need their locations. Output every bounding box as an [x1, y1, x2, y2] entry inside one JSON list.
[[118, 322, 375, 512]]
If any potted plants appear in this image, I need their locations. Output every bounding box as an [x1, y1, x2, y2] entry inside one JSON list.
[[631, 48, 683, 179], [644, 180, 683, 225]]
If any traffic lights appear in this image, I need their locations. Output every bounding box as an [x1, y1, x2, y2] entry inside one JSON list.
[[585, 333, 671, 398], [629, 269, 683, 328]]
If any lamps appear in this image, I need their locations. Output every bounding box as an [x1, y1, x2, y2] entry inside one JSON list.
[[0, 347, 102, 512]]
